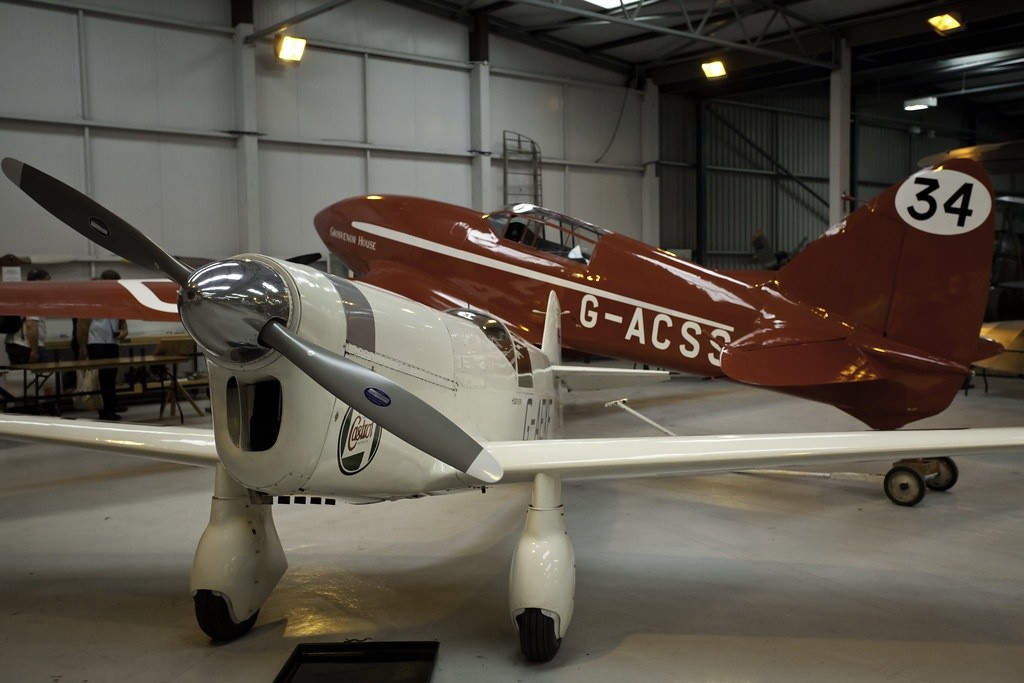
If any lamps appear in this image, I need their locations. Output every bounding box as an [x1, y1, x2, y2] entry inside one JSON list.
[[926, 7, 967, 37], [272, 34, 307, 62]]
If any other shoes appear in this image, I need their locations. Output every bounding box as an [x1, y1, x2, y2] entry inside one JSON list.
[[99, 414, 121, 421], [113, 406, 128, 413]]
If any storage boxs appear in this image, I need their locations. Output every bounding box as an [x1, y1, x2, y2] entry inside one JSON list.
[[2, 266, 28, 282]]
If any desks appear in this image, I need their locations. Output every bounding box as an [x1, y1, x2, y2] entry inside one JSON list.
[[0, 354, 195, 424]]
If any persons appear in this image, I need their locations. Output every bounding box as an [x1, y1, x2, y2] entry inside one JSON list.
[[5, 268, 51, 364], [72, 269, 128, 420]]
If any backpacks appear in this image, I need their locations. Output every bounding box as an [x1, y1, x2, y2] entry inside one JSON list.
[[0, 315, 27, 341]]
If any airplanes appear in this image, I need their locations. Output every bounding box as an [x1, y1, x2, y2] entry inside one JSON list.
[[3, 143, 1023, 512], [1, 157, 1024, 683]]
